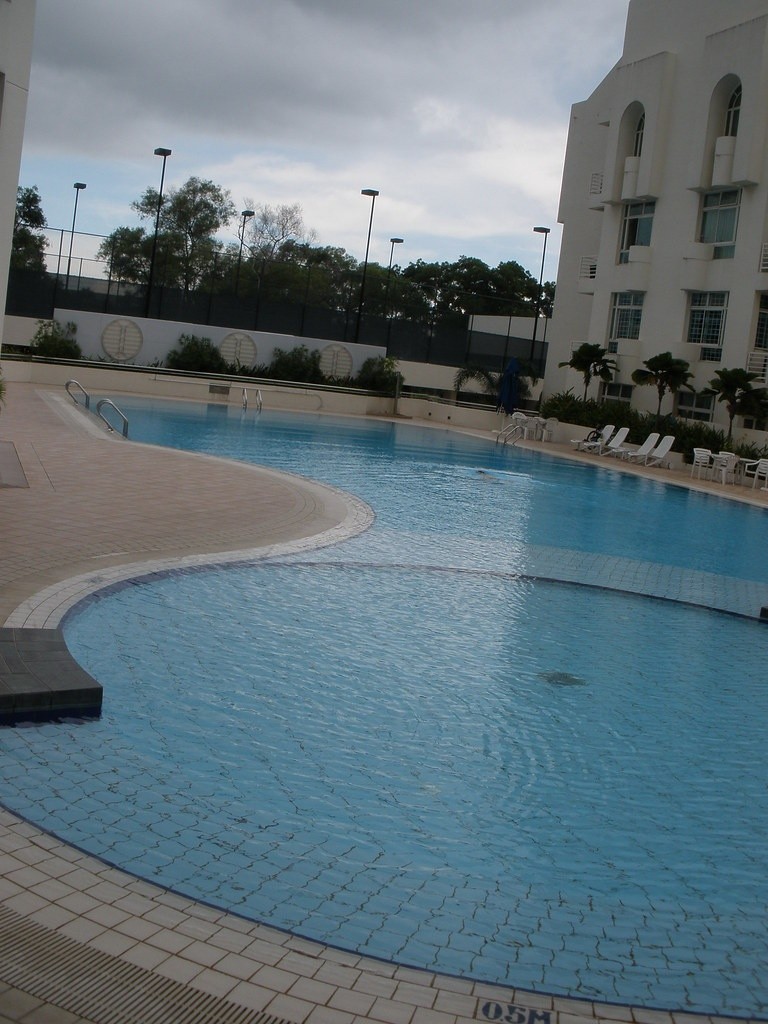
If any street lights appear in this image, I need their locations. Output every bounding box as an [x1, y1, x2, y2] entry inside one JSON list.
[[229, 210, 255, 327], [383, 237, 403, 320], [144, 146, 171, 317], [65, 181, 87, 289], [528, 226, 550, 375], [354, 190, 379, 344]]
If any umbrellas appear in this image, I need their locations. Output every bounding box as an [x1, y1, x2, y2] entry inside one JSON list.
[[495, 355, 522, 424]]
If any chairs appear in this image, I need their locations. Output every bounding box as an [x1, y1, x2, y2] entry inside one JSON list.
[[629, 433, 661, 465], [743, 459, 768, 489], [598, 427, 629, 456], [583, 425, 615, 454], [642, 435, 675, 469], [719, 452, 741, 486], [690, 448, 711, 481], [511, 411, 559, 442]]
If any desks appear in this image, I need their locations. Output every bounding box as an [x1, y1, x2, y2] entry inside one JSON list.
[[707, 453, 732, 483], [571, 439, 583, 450], [740, 458, 757, 462], [613, 447, 634, 459]]
